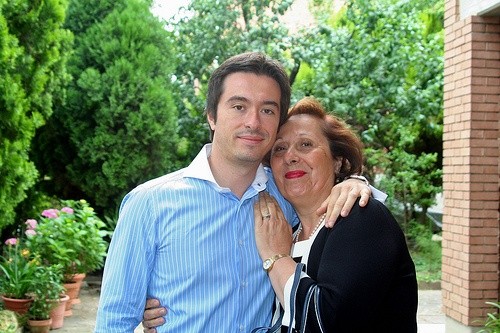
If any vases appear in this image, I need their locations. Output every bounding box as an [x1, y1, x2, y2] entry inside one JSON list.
[[1, 273, 86, 333]]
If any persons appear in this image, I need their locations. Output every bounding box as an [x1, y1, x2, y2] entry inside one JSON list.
[[96, 51, 389, 333], [140, 98, 420, 333]]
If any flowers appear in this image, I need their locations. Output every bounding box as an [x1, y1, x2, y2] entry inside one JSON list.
[[0, 199, 110, 320]]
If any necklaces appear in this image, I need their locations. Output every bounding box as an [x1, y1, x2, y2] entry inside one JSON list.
[[293, 214, 326, 242]]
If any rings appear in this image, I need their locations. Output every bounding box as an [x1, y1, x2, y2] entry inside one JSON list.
[[260, 214, 272, 220]]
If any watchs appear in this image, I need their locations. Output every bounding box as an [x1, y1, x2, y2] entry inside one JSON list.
[[262, 253, 289, 275]]
